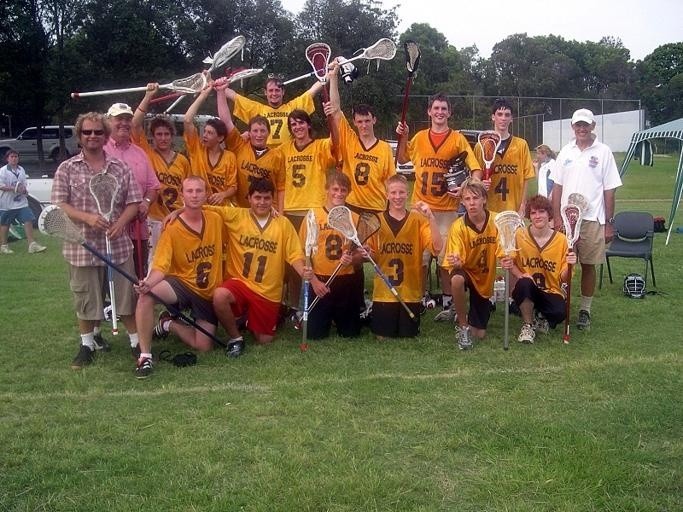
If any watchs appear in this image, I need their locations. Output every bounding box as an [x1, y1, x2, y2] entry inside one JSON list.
[[606, 218, 616, 225]]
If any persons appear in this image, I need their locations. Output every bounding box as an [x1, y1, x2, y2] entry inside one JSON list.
[[434, 177, 517, 350], [548, 107, 623, 331], [0, 149, 48, 254], [395, 92, 484, 315], [510, 194, 578, 344], [473, 99, 536, 312], [51, 57, 396, 379], [355, 173, 444, 338], [532, 144, 557, 233]]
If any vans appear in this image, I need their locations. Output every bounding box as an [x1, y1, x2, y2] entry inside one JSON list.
[[453, 130, 487, 151], [134, 111, 225, 156]]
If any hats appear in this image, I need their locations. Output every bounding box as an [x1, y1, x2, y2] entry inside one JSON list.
[[570, 109, 596, 128], [106, 103, 135, 119]]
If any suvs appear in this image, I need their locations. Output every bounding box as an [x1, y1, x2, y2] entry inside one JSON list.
[[382, 138, 417, 180], [0, 124, 83, 164]]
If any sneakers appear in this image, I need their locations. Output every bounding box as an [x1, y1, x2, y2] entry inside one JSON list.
[[130, 343, 142, 360], [576, 309, 592, 330], [535, 314, 550, 333], [0, 242, 48, 254], [456, 326, 473, 350], [153, 312, 171, 340], [518, 324, 536, 344], [359, 298, 374, 320], [434, 304, 457, 323], [94, 333, 111, 351], [225, 337, 245, 359], [280, 303, 303, 330], [134, 354, 154, 379], [419, 291, 431, 315], [70, 344, 95, 370]]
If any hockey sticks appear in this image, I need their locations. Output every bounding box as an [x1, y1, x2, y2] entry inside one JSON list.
[[89, 174, 118, 336], [277, 38, 396, 88], [395, 40, 421, 168], [328, 206, 415, 319], [294, 211, 381, 329], [478, 131, 501, 181], [71, 36, 263, 115], [302, 209, 315, 351], [559, 192, 589, 232], [305, 42, 340, 168], [38, 205, 230, 350], [561, 205, 583, 343], [493, 211, 521, 348]]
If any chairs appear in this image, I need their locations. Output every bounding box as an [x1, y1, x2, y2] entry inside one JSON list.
[[608, 209, 657, 288]]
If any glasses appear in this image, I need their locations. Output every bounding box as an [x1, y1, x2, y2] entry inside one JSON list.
[[79, 129, 105, 135]]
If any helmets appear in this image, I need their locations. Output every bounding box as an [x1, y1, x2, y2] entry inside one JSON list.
[[622, 273, 646, 300]]
